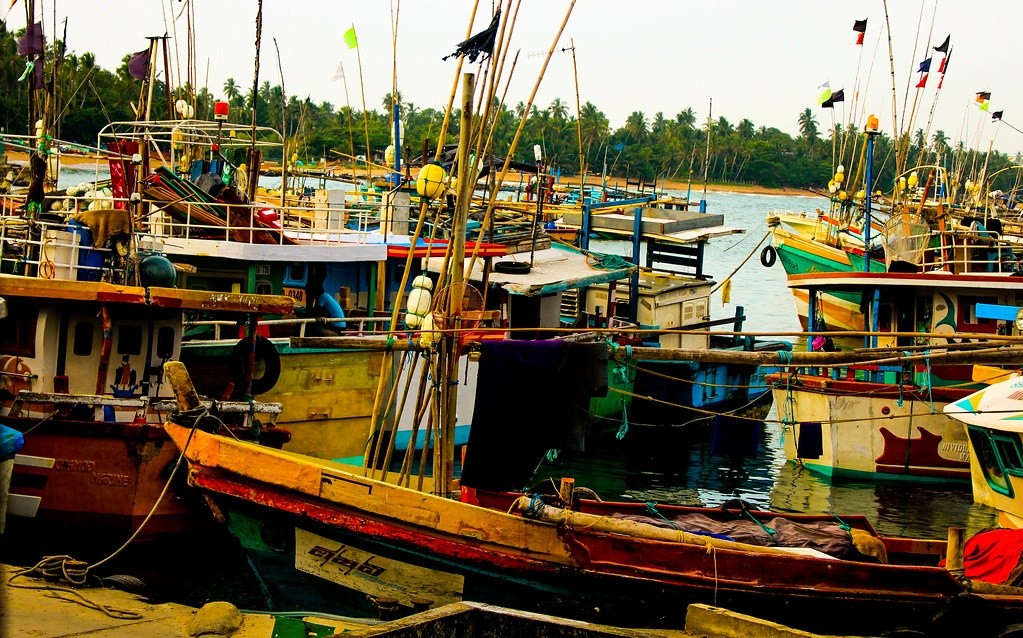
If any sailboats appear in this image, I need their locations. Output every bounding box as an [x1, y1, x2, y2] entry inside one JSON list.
[[0, 0, 1023, 637]]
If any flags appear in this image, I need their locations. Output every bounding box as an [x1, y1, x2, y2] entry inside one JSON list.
[[816, 80, 845, 108], [852, 18, 867, 46], [441, 7, 503, 64], [975, 90, 1003, 123], [128, 47, 150, 82], [916, 35, 951, 90], [343, 25, 358, 49], [331, 62, 344, 82], [19, 20, 44, 90]]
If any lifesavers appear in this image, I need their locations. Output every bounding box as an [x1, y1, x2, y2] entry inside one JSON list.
[[232, 336, 281, 394], [761, 245, 777, 267]]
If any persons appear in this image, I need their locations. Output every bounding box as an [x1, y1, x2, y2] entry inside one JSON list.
[[305, 281, 346, 336]]
